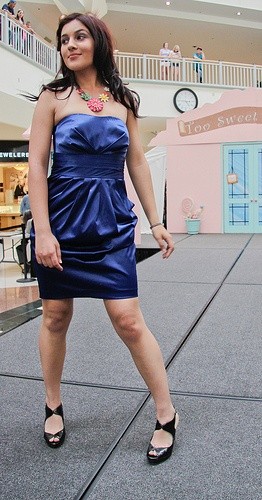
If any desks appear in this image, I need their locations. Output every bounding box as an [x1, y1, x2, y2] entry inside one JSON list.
[[0, 232, 26, 273]]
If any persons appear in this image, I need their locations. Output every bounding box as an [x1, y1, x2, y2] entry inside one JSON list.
[[192, 48, 205, 83], [27, 13, 180, 463], [20, 194, 32, 225], [0, 0, 34, 56], [159, 43, 181, 81]]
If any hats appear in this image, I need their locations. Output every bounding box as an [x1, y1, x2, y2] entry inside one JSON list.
[[10, 0, 16, 3]]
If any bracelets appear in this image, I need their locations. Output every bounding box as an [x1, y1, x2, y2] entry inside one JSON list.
[[150, 223, 162, 230]]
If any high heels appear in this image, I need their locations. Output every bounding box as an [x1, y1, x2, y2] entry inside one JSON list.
[[44, 401, 66, 449], [147, 411, 177, 465]]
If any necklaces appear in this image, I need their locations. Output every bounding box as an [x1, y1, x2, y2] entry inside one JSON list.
[[74, 85, 110, 112]]
